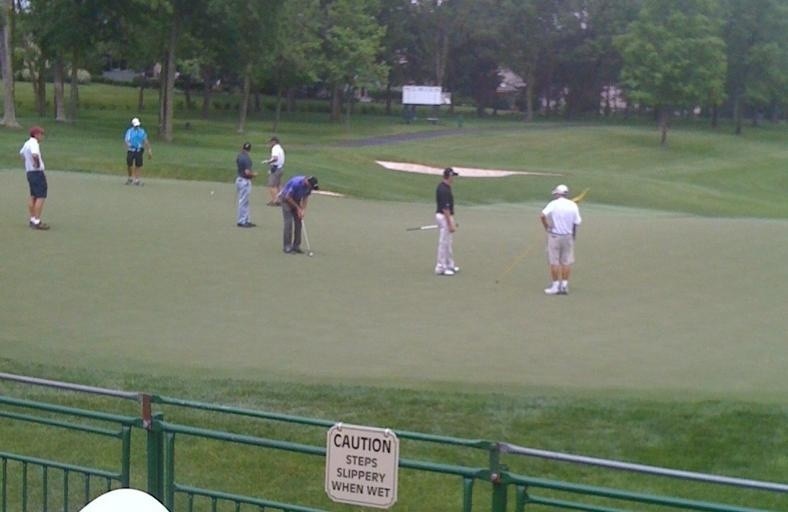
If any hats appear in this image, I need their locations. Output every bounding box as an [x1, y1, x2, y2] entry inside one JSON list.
[[30, 127, 45, 137], [307, 176, 320, 190], [551, 184, 569, 195], [444, 168, 458, 176], [132, 117, 141, 127]]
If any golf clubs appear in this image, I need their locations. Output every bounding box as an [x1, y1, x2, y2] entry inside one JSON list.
[[300, 216, 316, 256]]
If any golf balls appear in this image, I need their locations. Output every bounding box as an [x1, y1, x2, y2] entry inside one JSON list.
[[210, 189, 216, 195]]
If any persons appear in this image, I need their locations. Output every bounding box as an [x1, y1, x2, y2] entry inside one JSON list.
[[125, 117, 152, 185], [277, 175, 320, 255], [540, 184, 583, 295], [434, 167, 460, 276], [235, 142, 258, 227], [262, 136, 285, 206], [20, 127, 50, 230]]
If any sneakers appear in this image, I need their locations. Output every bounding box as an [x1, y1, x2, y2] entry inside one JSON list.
[[237, 200, 305, 254], [134, 181, 144, 187], [31, 220, 50, 229], [435, 269, 454, 275], [125, 179, 133, 185], [544, 286, 560, 294], [560, 284, 568, 294], [453, 267, 459, 272]]
